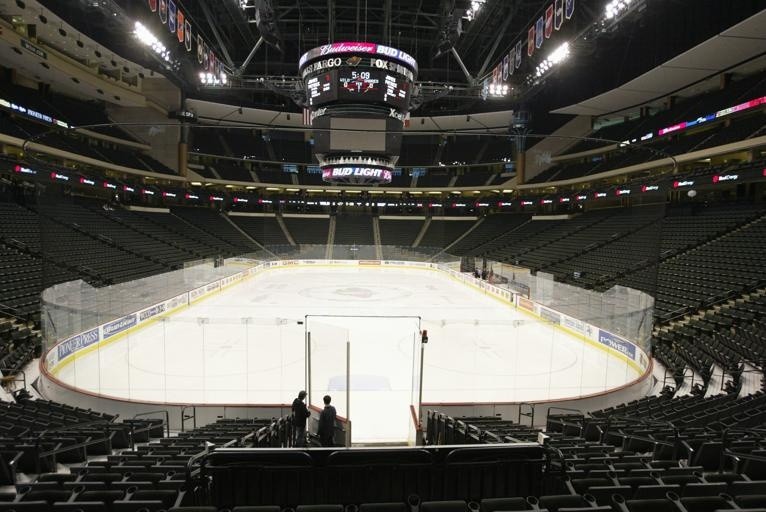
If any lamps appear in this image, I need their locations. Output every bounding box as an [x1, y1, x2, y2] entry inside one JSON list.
[[269, 103, 290, 124], [467, 116, 487, 129], [220, 107, 242, 122], [420, 115, 442, 129]]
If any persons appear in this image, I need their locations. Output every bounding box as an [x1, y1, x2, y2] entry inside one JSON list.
[[317, 395, 336, 446], [292, 391, 311, 447]]
